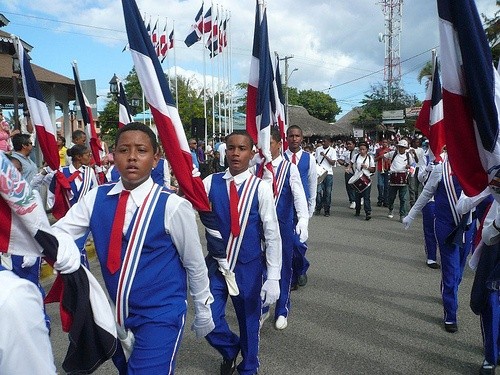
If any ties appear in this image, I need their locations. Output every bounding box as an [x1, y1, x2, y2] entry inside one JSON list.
[[67, 171, 80, 183], [105, 189, 130, 274], [292, 153, 296, 165], [265, 162, 278, 196], [230, 180, 241, 236]]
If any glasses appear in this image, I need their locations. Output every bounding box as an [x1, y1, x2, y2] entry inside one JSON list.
[[29, 141, 32, 145], [84, 152, 92, 157]]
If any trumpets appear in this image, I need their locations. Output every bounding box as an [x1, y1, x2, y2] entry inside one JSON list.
[[380, 158, 384, 174]]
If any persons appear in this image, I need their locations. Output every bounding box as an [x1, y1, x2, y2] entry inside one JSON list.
[[50, 122, 216, 375], [0, 109, 500, 375], [191, 130, 282, 375]]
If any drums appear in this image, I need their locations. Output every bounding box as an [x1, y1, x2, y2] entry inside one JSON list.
[[388, 170, 409, 186], [316, 162, 328, 185], [348, 170, 372, 194]]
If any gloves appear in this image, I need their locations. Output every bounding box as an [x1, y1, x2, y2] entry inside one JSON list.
[[21, 255, 37, 269], [43, 165, 58, 174], [191, 162, 202, 177], [402, 216, 413, 231], [296, 218, 309, 243], [191, 317, 215, 339], [425, 161, 435, 172], [458, 198, 473, 213], [53, 232, 81, 274], [260, 279, 280, 307]]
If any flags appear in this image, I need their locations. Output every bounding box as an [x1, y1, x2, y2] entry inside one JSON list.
[[121, 0, 211, 212], [414, 55, 447, 162], [71, 60, 105, 185], [0, 150, 118, 375], [184, 3, 227, 59], [244, 0, 287, 167], [121, 14, 175, 64], [437, 0, 500, 196], [117, 78, 135, 130], [18, 36, 74, 220]]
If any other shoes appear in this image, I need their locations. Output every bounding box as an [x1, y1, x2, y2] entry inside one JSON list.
[[315, 208, 330, 217], [290, 272, 307, 290], [259, 309, 288, 330], [355, 210, 372, 220], [219, 342, 241, 375], [478, 359, 496, 375], [387, 209, 405, 224], [445, 322, 458, 333], [349, 197, 364, 209], [426, 259, 440, 269], [376, 200, 389, 207]]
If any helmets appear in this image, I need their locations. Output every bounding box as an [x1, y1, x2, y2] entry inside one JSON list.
[[396, 140, 409, 148]]
